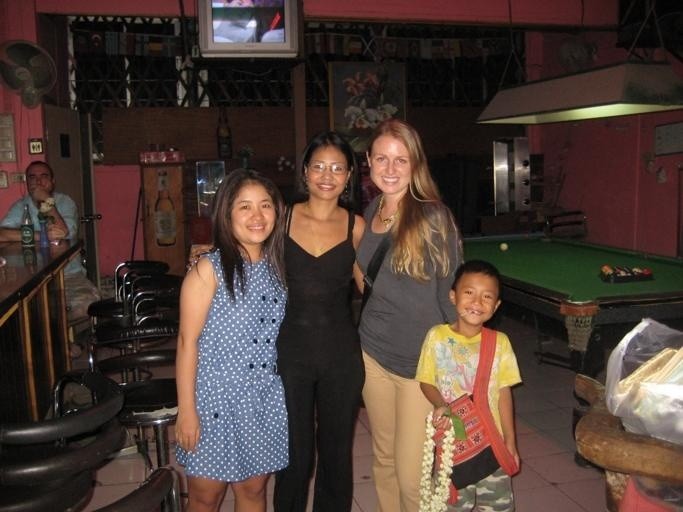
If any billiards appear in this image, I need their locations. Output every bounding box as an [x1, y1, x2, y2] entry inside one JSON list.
[[501, 243, 508, 250], [600, 266, 651, 277]]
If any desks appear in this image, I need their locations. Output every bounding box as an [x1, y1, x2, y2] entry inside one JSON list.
[[462, 232, 683, 468]]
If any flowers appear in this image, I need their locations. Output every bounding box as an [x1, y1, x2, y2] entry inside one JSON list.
[[37, 198, 56, 224], [343, 72, 399, 152]]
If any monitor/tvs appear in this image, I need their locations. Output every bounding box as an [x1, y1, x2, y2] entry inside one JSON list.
[[198, 0, 305, 58]]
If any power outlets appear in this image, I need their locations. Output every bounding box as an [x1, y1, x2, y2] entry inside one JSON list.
[[12, 174, 26, 182]]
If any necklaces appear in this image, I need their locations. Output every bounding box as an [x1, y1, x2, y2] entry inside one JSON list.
[[378, 194, 395, 228]]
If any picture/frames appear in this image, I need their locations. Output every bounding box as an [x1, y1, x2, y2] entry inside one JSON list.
[[328, 62, 409, 156], [654, 121, 683, 156]]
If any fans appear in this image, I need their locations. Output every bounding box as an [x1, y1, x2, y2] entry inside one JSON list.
[[0, 40, 58, 109]]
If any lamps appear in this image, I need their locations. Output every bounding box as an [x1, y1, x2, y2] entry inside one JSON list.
[[472, 0, 683, 124]]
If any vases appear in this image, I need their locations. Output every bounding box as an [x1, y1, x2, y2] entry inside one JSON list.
[[39, 220, 50, 248]]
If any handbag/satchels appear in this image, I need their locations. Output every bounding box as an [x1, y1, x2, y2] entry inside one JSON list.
[[433, 393, 519, 504]]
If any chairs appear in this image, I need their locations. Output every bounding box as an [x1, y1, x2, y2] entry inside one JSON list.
[[573, 374, 683, 512]]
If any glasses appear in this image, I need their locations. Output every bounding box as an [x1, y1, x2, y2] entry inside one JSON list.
[[308, 163, 346, 174]]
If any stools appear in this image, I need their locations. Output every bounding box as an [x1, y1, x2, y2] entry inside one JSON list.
[[0, 239, 184, 511]]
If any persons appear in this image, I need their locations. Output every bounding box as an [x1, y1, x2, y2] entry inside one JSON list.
[[0, 161, 102, 359], [415, 261, 524, 512], [174, 166, 292, 511], [357, 119, 465, 512], [188, 131, 366, 512]]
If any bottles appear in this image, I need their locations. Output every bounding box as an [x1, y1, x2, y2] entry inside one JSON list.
[[215, 107, 233, 162], [19, 204, 34, 250], [153, 169, 177, 249]]
